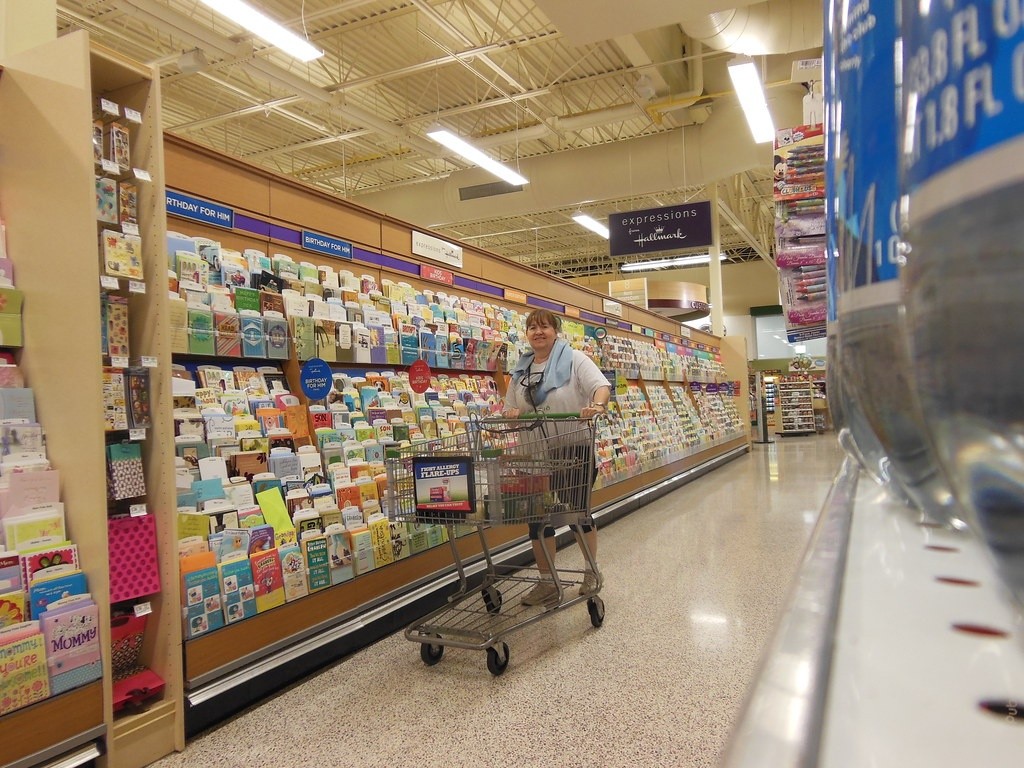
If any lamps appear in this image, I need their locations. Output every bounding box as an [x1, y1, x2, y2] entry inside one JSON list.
[[200, 0, 325, 64], [570, 212, 609, 240], [718, 253, 729, 261], [426, 122, 530, 186], [622, 254, 708, 272], [727, 54, 776, 144]]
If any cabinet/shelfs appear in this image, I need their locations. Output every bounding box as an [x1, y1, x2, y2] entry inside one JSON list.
[[0, 28, 833, 768]]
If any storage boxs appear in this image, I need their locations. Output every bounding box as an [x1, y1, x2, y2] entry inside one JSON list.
[[484, 494, 546, 523], [499, 456, 552, 494]]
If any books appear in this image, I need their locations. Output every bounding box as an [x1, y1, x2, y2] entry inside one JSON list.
[[0, 219, 527, 768], [556, 318, 745, 489]]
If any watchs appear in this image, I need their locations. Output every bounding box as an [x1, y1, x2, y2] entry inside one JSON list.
[[592, 402, 606, 411]]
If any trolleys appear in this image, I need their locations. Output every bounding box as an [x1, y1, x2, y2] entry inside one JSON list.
[[384, 407, 606, 673]]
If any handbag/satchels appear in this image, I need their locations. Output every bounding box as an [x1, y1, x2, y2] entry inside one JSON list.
[[593, 468, 598, 487]]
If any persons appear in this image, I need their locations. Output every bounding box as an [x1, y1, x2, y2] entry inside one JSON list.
[[501, 307, 612, 606]]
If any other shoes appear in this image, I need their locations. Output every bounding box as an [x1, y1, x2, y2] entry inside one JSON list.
[[579, 571, 604, 595], [521, 581, 557, 606]]
